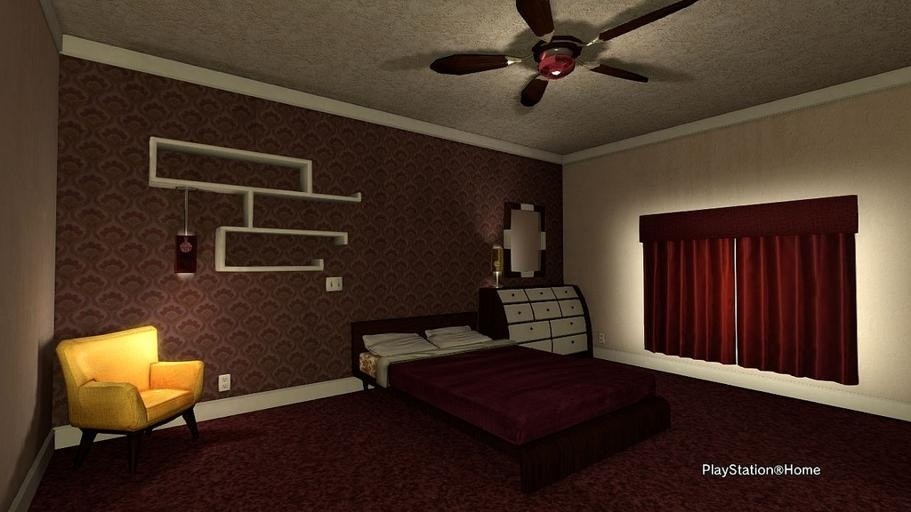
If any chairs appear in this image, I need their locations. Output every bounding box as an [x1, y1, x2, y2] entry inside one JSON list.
[[56, 321, 206, 481]]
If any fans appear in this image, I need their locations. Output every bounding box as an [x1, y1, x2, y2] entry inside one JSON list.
[[429, 1, 698, 111]]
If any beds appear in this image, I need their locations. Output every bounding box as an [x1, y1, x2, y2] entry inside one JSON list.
[[351, 312, 673, 495]]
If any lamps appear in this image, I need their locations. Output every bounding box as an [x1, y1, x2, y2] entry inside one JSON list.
[[174, 185, 199, 281], [537, 47, 577, 81]]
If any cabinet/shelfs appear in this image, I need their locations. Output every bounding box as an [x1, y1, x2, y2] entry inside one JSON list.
[[480, 283, 594, 362], [144, 135, 363, 274]]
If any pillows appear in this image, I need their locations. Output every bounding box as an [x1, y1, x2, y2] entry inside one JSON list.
[[360, 325, 494, 360]]
